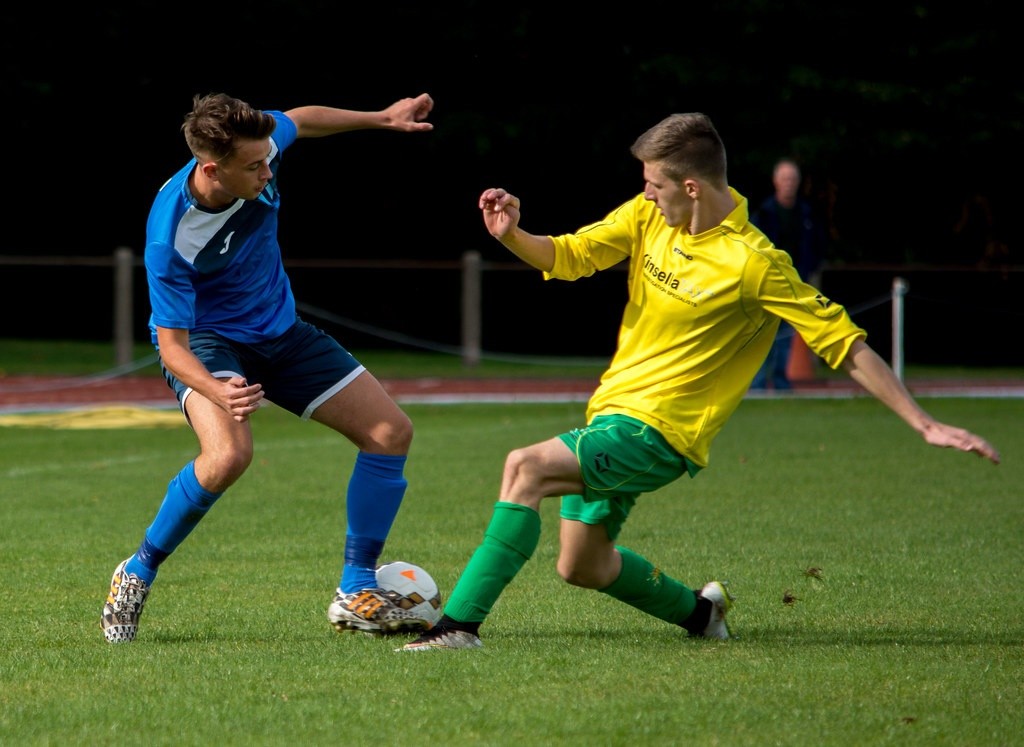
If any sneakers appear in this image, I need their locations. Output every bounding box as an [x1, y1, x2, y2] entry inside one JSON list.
[[389, 625, 485, 650], [99, 552, 150, 641], [691, 581, 736, 638], [328, 587, 430, 637]]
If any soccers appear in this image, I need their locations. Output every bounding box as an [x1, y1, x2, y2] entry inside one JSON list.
[[375, 561, 442, 629]]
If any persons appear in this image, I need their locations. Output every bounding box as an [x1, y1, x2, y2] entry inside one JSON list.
[[395, 112, 1001, 652], [97, 93, 433, 641], [750, 159, 823, 389]]
